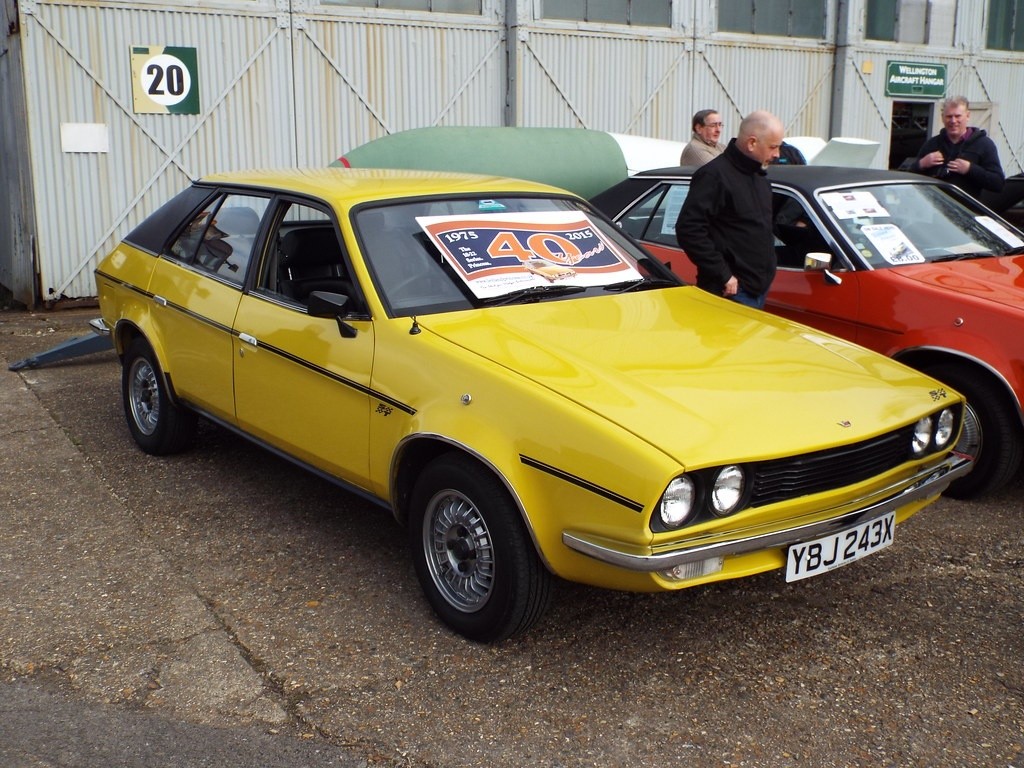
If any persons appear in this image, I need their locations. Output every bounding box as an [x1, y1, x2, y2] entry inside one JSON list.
[[680, 109, 727, 167], [675, 109, 785, 310], [909, 96, 1006, 202]]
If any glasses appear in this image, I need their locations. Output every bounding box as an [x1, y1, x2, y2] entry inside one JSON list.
[[703, 122, 724, 130]]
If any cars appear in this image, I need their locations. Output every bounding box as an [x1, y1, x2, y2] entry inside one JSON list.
[[87, 168, 974, 648], [586, 163, 1024, 500]]
